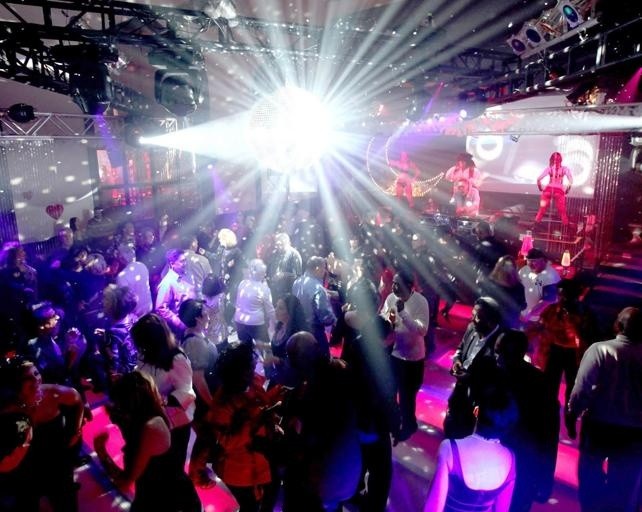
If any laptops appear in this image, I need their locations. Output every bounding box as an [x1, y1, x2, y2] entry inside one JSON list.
[[439, 203, 456, 216]]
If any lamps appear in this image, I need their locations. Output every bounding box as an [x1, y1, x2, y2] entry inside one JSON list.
[[505, 0, 595, 57]]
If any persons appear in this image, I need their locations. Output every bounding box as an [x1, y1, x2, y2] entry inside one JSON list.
[[444, 151, 482, 216], [1, 196, 642, 511], [454, 178, 480, 219], [388, 149, 422, 209], [530, 152, 572, 237]]
